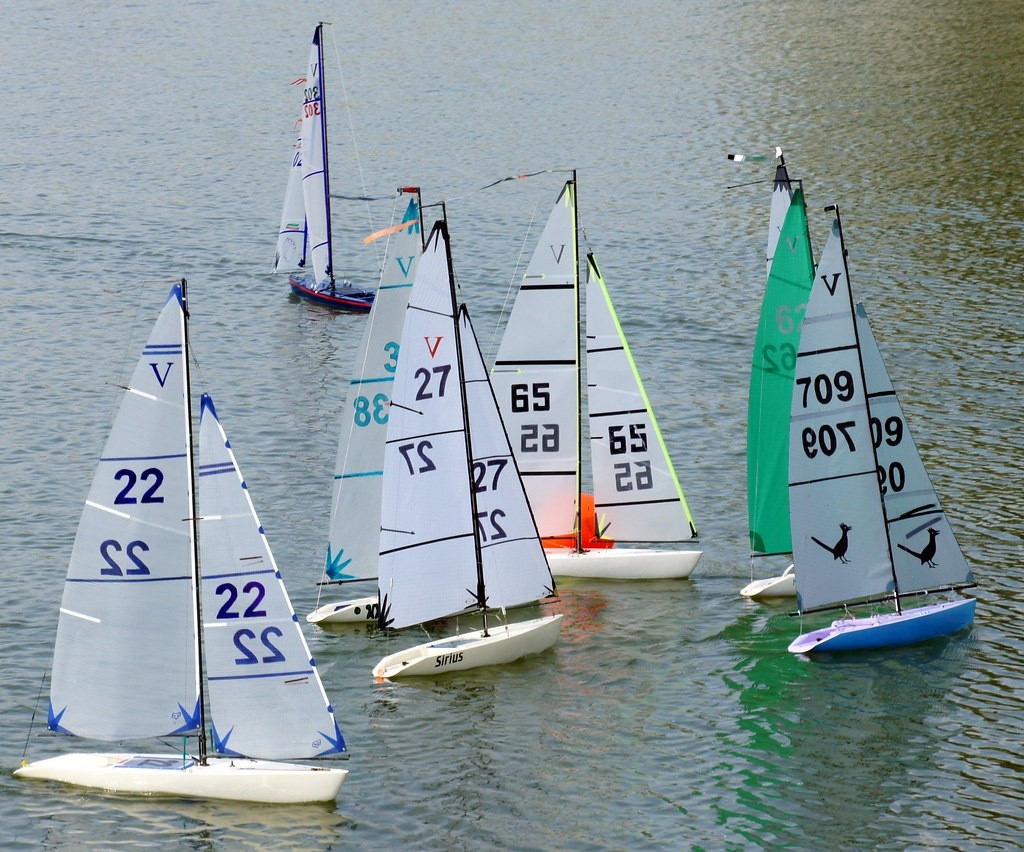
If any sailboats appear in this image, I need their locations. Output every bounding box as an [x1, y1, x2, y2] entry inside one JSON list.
[[487, 165, 705, 583], [271, 18, 380, 316], [786, 201, 978, 657], [740, 148, 817, 600], [15, 274, 351, 808], [368, 200, 562, 683], [306, 184, 429, 626]]
